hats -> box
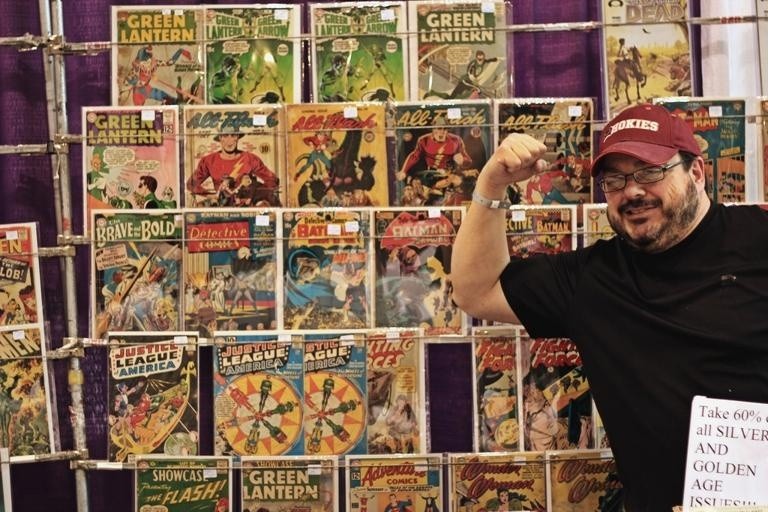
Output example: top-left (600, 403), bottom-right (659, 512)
top-left (590, 104), bottom-right (701, 177)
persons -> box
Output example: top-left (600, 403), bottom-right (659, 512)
top-left (449, 101), bottom-right (768, 512)
top-left (0, 285), bottom-right (39, 326)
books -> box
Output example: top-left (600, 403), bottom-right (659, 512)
top-left (81, 0), bottom-right (745, 512)
top-left (0, 321), bottom-right (63, 466)
top-left (0, 219), bottom-right (45, 327)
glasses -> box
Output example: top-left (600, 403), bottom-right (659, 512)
top-left (596, 159), bottom-right (684, 193)
